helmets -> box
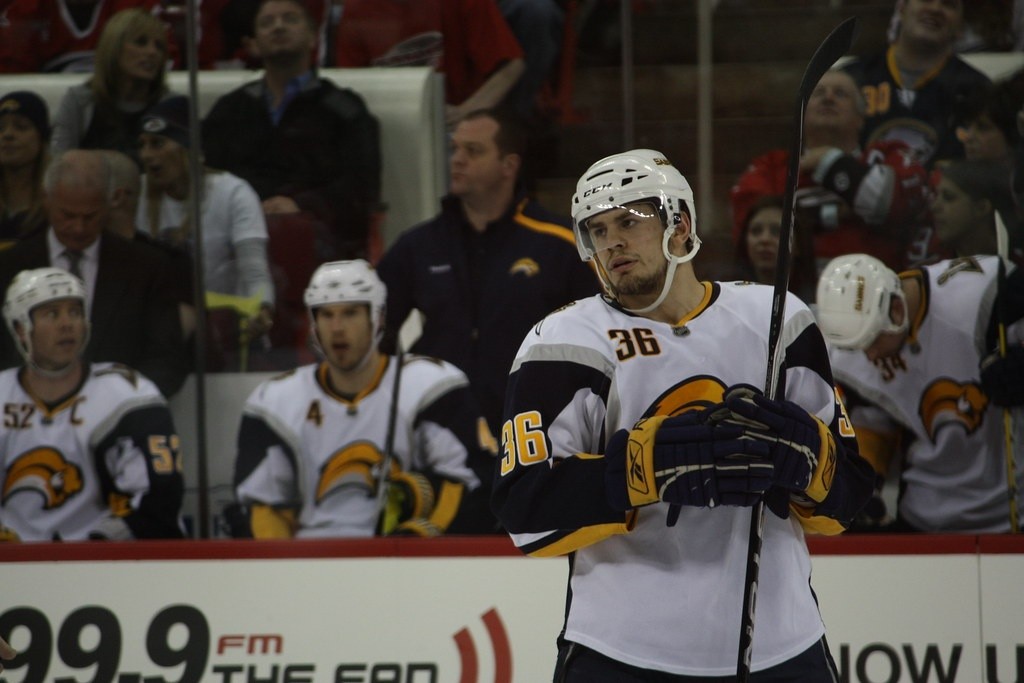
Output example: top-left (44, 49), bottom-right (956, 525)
top-left (816, 254), bottom-right (909, 349)
top-left (3, 266), bottom-right (90, 360)
top-left (571, 149), bottom-right (694, 261)
top-left (303, 259), bottom-right (386, 337)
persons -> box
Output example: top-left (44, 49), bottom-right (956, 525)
top-left (727, 0), bottom-right (1024, 537)
top-left (233, 259), bottom-right (497, 543)
top-left (0, 268), bottom-right (184, 546)
top-left (490, 149), bottom-right (874, 683)
top-left (0, 0), bottom-right (326, 393)
top-left (376, 109), bottom-right (601, 417)
top-left (201, 0), bottom-right (383, 370)
top-left (333, 0), bottom-right (526, 143)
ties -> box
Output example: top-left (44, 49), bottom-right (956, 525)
top-left (63, 249), bottom-right (81, 280)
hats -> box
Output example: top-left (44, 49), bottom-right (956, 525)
top-left (137, 95), bottom-right (190, 145)
top-left (0, 91), bottom-right (48, 137)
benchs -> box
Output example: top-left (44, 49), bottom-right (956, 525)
top-left (0, 48), bottom-right (1024, 540)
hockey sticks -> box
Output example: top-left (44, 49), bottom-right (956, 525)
top-left (992, 209), bottom-right (1019, 532)
top-left (736, 16), bottom-right (858, 682)
top-left (374, 307), bottom-right (424, 540)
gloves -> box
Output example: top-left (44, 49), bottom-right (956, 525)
top-left (817, 150), bottom-right (891, 218)
top-left (979, 322), bottom-right (1024, 406)
top-left (605, 401), bottom-right (774, 527)
top-left (716, 384), bottom-right (836, 506)
